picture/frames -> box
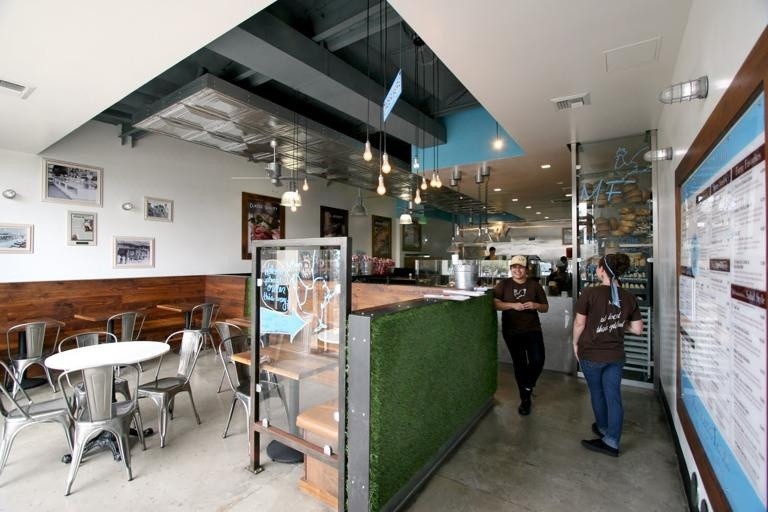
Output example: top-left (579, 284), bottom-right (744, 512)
top-left (66, 209), bottom-right (99, 247)
top-left (368, 214), bottom-right (392, 260)
top-left (0, 222), bottom-right (34, 255)
top-left (238, 190), bottom-right (288, 262)
top-left (401, 218), bottom-right (423, 253)
top-left (142, 195), bottom-right (174, 224)
top-left (318, 204), bottom-right (350, 250)
top-left (39, 156), bottom-right (104, 209)
top-left (109, 234), bottom-right (156, 269)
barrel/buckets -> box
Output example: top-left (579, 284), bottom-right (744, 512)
top-left (352, 261), bottom-right (361, 277)
top-left (453, 263), bottom-right (479, 291)
top-left (360, 260), bottom-right (376, 275)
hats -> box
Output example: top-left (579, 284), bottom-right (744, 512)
top-left (509, 255), bottom-right (527, 267)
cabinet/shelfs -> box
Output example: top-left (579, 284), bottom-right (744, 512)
top-left (578, 201), bottom-right (652, 307)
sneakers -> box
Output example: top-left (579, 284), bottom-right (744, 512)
top-left (592, 421), bottom-right (605, 437)
top-left (518, 386), bottom-right (533, 416)
top-left (581, 438), bottom-right (619, 458)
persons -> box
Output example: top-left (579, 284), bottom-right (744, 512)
top-left (493, 255), bottom-right (549, 416)
top-left (485, 247), bottom-right (497, 260)
top-left (546, 256), bottom-right (573, 296)
top-left (572, 254), bottom-right (643, 458)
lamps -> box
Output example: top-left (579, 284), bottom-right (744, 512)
top-left (656, 72), bottom-right (710, 106)
top-left (399, 201), bottom-right (428, 225)
top-left (494, 188), bottom-right (502, 192)
top-left (450, 166), bottom-right (462, 242)
top-left (279, 116), bottom-right (302, 213)
top-left (476, 162), bottom-right (490, 237)
top-left (469, 209), bottom-right (473, 223)
top-left (642, 146), bottom-right (673, 163)
top-left (350, 188), bottom-right (367, 217)
top-left (302, 122), bottom-right (309, 192)
top-left (493, 138), bottom-right (503, 149)
top-left (363, 2), bottom-right (373, 161)
top-left (381, 2), bottom-right (392, 174)
top-left (413, 34), bottom-right (443, 205)
top-left (377, 2), bottom-right (387, 197)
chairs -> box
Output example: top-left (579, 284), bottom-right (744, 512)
top-left (219, 334), bottom-right (291, 457)
top-left (1, 358), bottom-right (75, 468)
top-left (1, 318), bottom-right (66, 401)
top-left (57, 331), bottom-right (131, 418)
top-left (164, 301), bottom-right (222, 356)
top-left (57, 361), bottom-right (147, 497)
top-left (107, 309), bottom-right (147, 343)
top-left (214, 321), bottom-right (251, 394)
